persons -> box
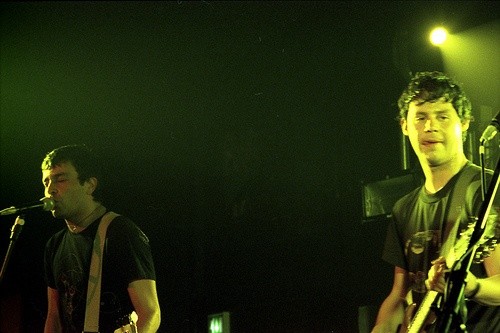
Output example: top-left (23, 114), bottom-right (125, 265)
top-left (36, 145), bottom-right (162, 333)
top-left (369, 69), bottom-right (500, 333)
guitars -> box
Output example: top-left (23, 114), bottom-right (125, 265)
top-left (397, 213), bottom-right (500, 333)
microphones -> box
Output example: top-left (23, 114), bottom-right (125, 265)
top-left (0, 197), bottom-right (56, 217)
top-left (478, 109), bottom-right (500, 142)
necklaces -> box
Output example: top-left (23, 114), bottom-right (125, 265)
top-left (64, 204), bottom-right (103, 231)
